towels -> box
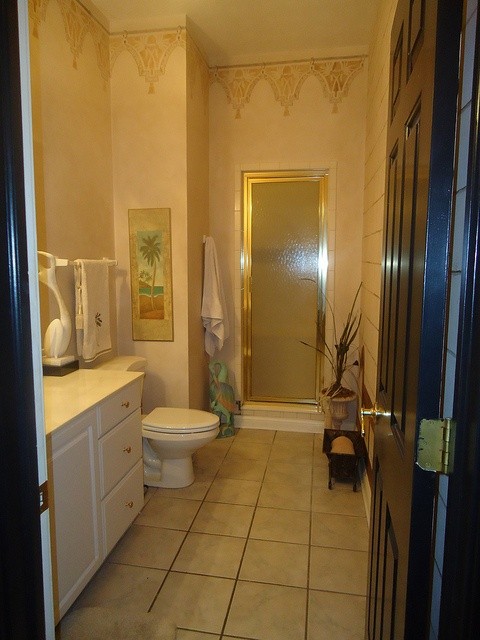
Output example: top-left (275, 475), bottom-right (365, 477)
top-left (74, 259), bottom-right (113, 363)
top-left (200, 235), bottom-right (230, 358)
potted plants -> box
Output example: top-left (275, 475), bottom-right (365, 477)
top-left (295, 277), bottom-right (363, 430)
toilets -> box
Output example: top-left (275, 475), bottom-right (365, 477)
top-left (94, 355), bottom-right (220, 489)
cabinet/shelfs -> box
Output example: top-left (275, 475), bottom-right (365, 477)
top-left (96, 381), bottom-right (144, 555)
top-left (46, 404), bottom-right (105, 629)
